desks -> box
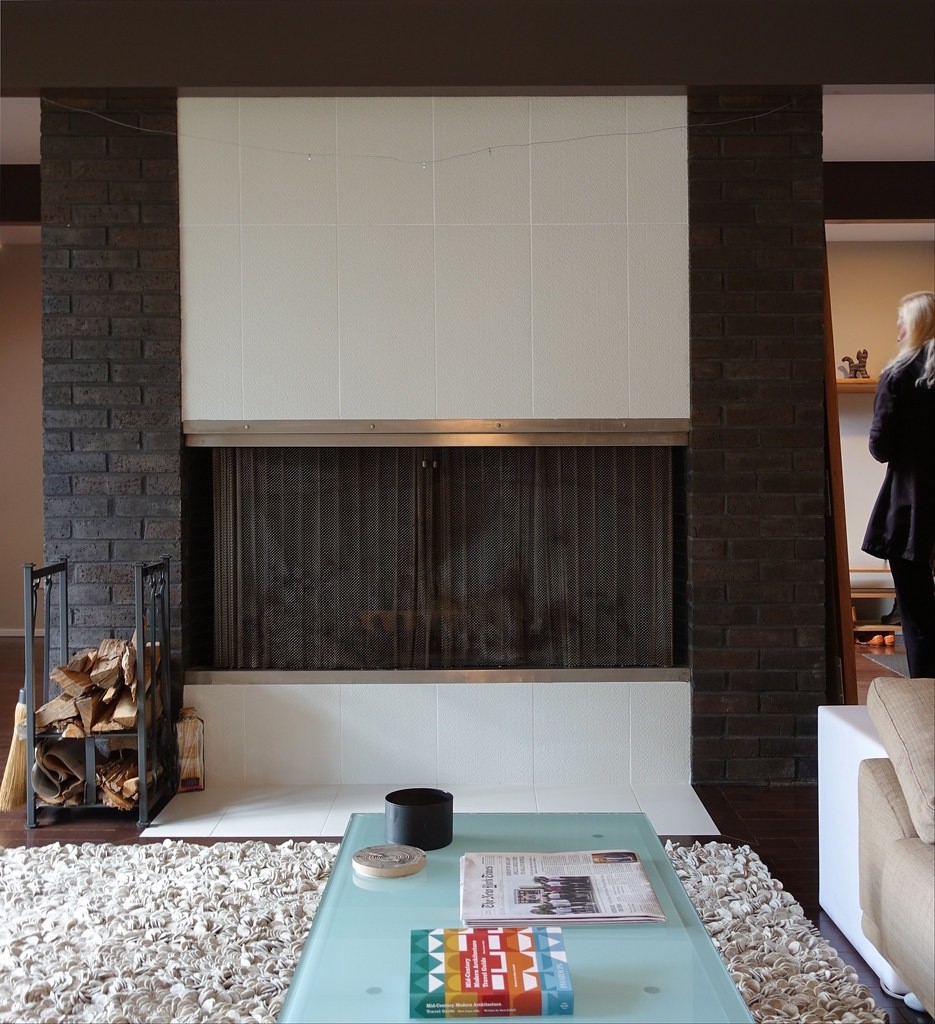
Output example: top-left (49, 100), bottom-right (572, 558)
top-left (279, 812), bottom-right (754, 1024)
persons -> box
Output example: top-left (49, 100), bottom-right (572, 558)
top-left (861, 292), bottom-right (935, 678)
top-left (593, 853), bottom-right (636, 863)
top-left (522, 876), bottom-right (595, 914)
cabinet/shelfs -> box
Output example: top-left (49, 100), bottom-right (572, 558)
top-left (21, 553), bottom-right (172, 828)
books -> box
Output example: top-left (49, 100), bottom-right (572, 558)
top-left (409, 926), bottom-right (574, 1019)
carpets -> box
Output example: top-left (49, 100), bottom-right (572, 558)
top-left (0, 839), bottom-right (890, 1024)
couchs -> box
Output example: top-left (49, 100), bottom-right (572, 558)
top-left (817, 678), bottom-right (935, 1020)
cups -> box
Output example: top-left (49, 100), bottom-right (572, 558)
top-left (383, 787), bottom-right (453, 851)
